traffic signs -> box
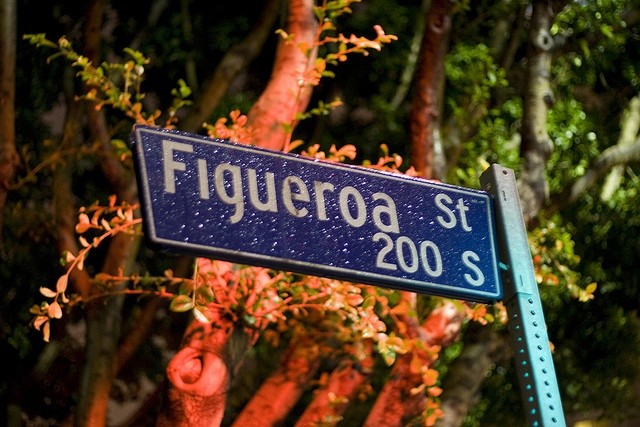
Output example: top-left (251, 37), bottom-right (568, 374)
top-left (131, 124), bottom-right (504, 303)
